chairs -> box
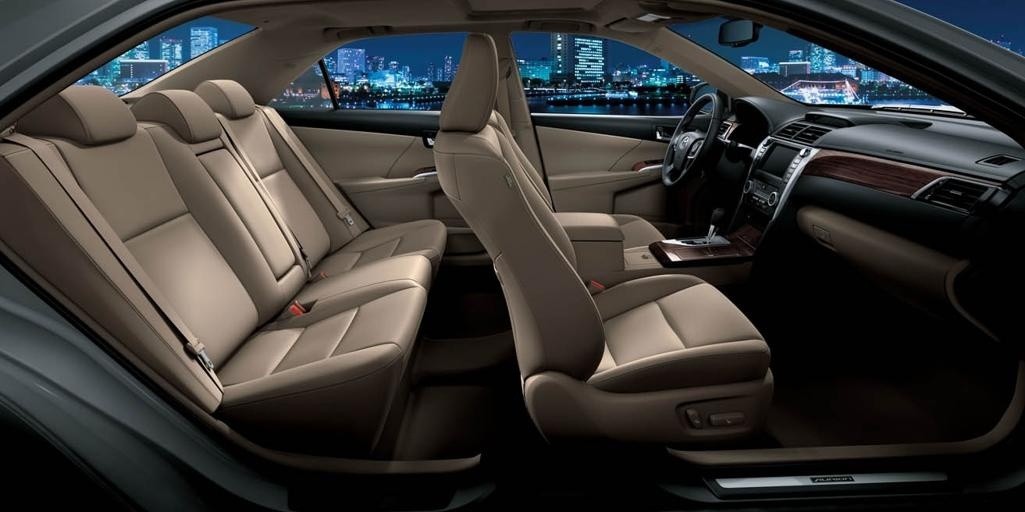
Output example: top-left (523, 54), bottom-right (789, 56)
top-left (194, 79), bottom-right (448, 274)
top-left (490, 32), bottom-right (696, 294)
top-left (430, 35), bottom-right (777, 448)
top-left (0, 82), bottom-right (428, 452)
top-left (130, 89), bottom-right (433, 332)
